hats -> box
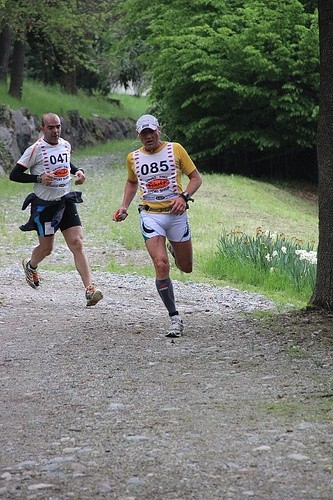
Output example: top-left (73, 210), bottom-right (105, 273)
top-left (135, 114), bottom-right (158, 134)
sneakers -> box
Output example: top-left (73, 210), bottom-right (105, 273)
top-left (22, 257), bottom-right (39, 289)
top-left (167, 242), bottom-right (173, 258)
top-left (85, 290), bottom-right (102, 306)
top-left (164, 316), bottom-right (183, 337)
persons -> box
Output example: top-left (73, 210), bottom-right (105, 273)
top-left (9, 113), bottom-right (103, 306)
top-left (114, 115), bottom-right (203, 336)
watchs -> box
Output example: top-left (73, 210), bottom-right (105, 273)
top-left (181, 192), bottom-right (190, 199)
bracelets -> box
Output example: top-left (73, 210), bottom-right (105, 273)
top-left (37, 176), bottom-right (41, 182)
